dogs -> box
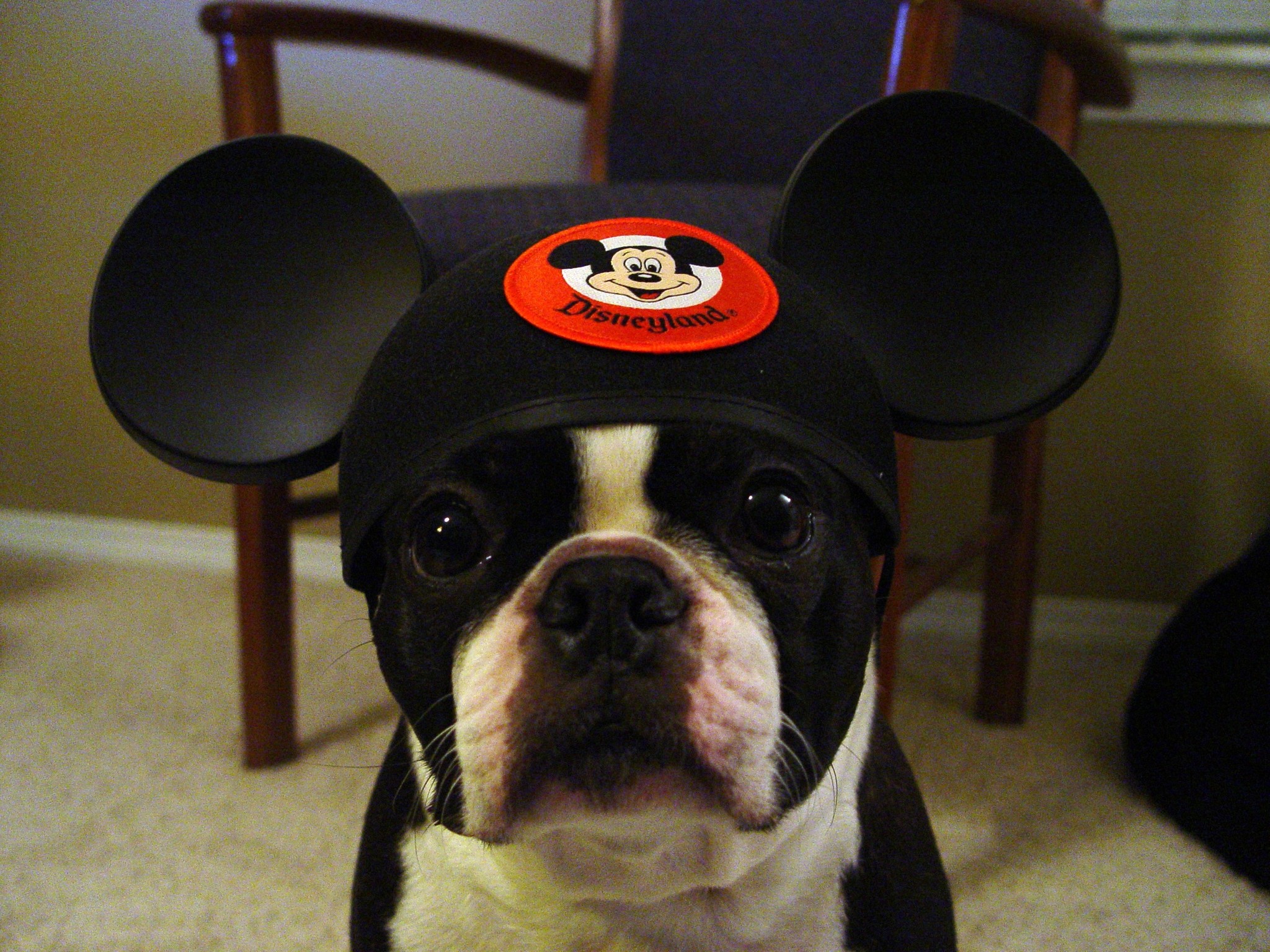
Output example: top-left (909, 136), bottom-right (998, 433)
top-left (348, 421), bottom-right (959, 952)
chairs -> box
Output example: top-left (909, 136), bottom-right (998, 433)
top-left (192, 2), bottom-right (1140, 782)
